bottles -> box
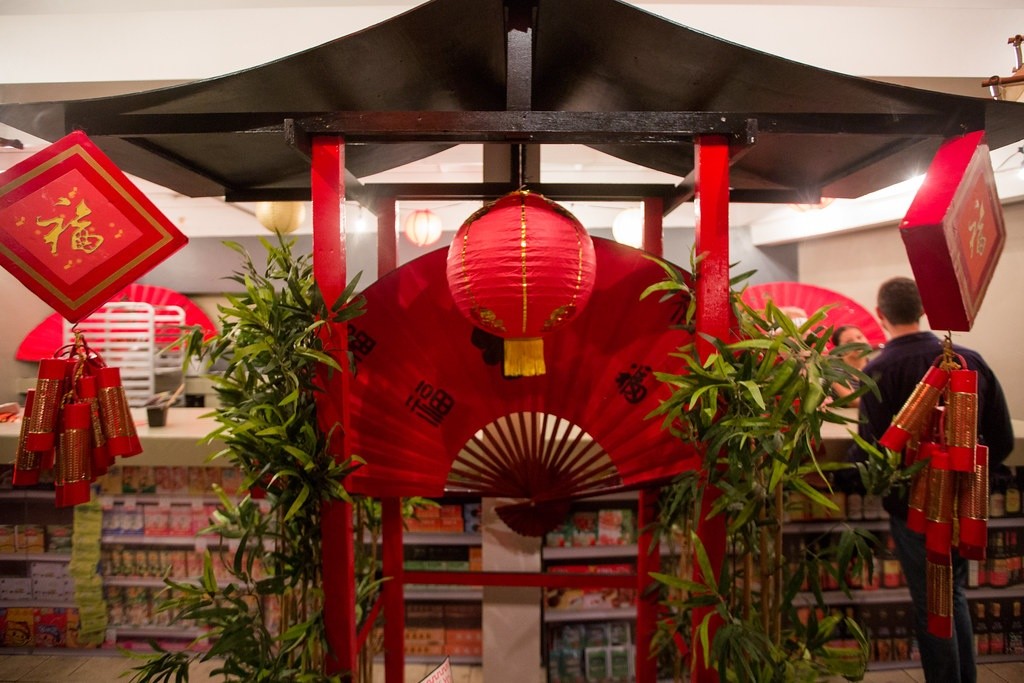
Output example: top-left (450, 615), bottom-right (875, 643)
top-left (769, 533), bottom-right (1024, 665)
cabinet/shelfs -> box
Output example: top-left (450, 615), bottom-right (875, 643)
top-left (0, 489), bottom-right (1024, 665)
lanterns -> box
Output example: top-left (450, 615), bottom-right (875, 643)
top-left (447, 191), bottom-right (596, 376)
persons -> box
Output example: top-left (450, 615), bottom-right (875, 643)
top-left (858, 277), bottom-right (1014, 683)
top-left (829, 326), bottom-right (871, 408)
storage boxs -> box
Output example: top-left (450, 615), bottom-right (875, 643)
top-left (0, 467), bottom-right (635, 682)
